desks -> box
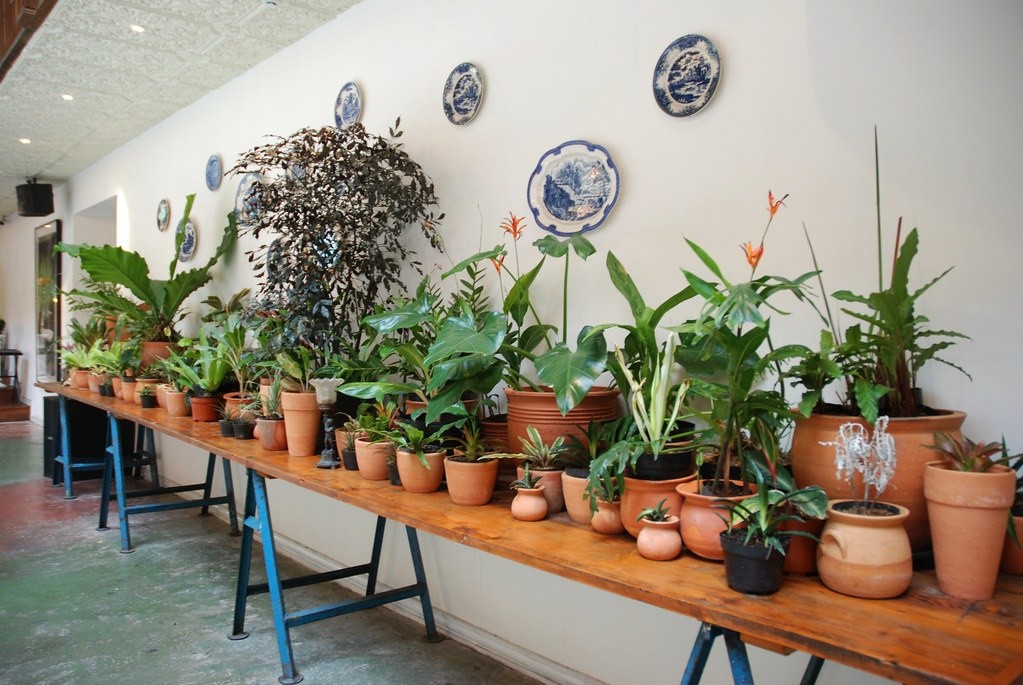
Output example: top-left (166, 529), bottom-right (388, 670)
top-left (0, 349), bottom-right (23, 406)
top-left (35, 382), bottom-right (1023, 685)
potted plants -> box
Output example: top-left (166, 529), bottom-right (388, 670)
top-left (41, 118), bottom-right (1023, 598)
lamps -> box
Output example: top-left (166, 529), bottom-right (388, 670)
top-left (15, 177), bottom-right (53, 218)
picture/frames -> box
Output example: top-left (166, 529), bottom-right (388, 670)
top-left (33, 220), bottom-right (62, 384)
